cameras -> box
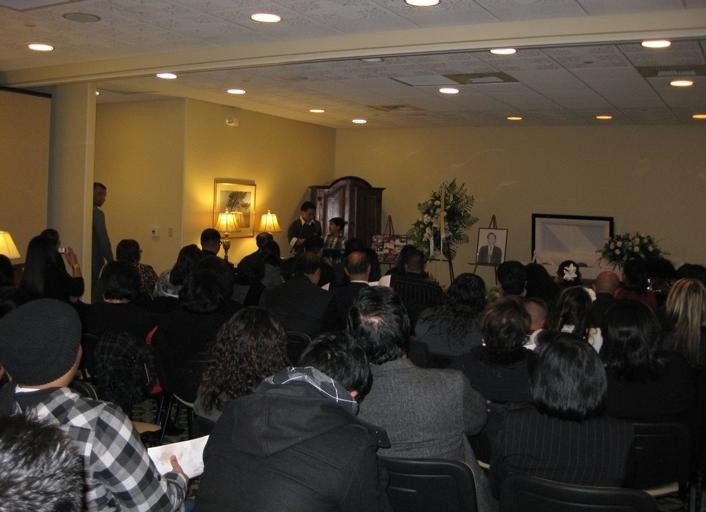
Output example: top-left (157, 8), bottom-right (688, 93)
top-left (57, 247), bottom-right (68, 254)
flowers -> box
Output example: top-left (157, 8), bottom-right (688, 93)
top-left (595, 232), bottom-right (671, 272)
top-left (407, 178), bottom-right (479, 263)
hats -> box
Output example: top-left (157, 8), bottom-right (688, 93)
top-left (1, 299), bottom-right (83, 386)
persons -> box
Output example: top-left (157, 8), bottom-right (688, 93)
top-left (324, 217), bottom-right (346, 276)
top-left (289, 201), bottom-right (323, 256)
top-left (1, 228), bottom-right (706, 511)
top-left (92, 183), bottom-right (115, 295)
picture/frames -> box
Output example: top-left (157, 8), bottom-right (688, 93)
top-left (477, 227), bottom-right (508, 264)
top-left (531, 213), bottom-right (614, 266)
top-left (213, 178), bottom-right (256, 240)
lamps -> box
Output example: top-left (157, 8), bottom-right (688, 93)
top-left (258, 210), bottom-right (282, 234)
top-left (0, 230), bottom-right (21, 259)
top-left (215, 210), bottom-right (241, 261)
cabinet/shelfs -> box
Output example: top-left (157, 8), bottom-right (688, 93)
top-left (309, 176), bottom-right (386, 244)
